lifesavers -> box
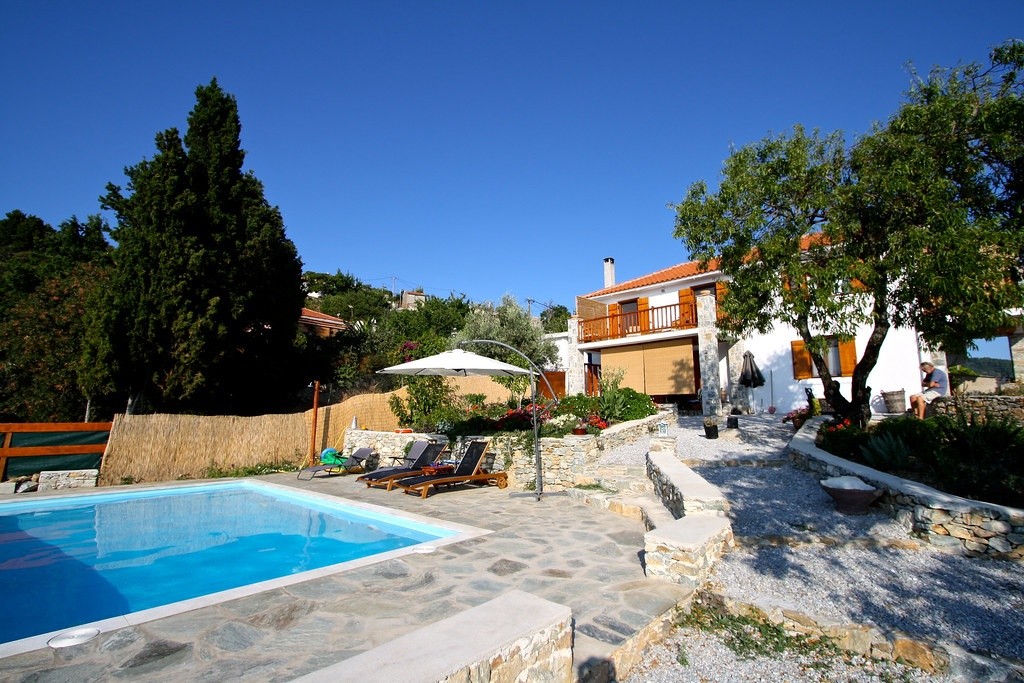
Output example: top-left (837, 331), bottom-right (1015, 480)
top-left (321, 448), bottom-right (336, 460)
top-left (396, 429), bottom-right (412, 433)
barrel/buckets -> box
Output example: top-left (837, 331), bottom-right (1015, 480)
top-left (880, 387), bottom-right (906, 413)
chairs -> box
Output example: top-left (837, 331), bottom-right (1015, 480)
top-left (368, 441), bottom-right (428, 474)
top-left (297, 448), bottom-right (375, 481)
top-left (355, 443), bottom-right (454, 490)
top-left (393, 441), bottom-right (508, 499)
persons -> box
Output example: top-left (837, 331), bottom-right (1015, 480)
top-left (910, 362), bottom-right (948, 418)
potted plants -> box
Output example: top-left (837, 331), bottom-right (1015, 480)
top-left (702, 416), bottom-right (719, 438)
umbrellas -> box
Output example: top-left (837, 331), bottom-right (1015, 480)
top-left (739, 350), bottom-right (766, 413)
top-left (376, 348), bottom-right (540, 378)
